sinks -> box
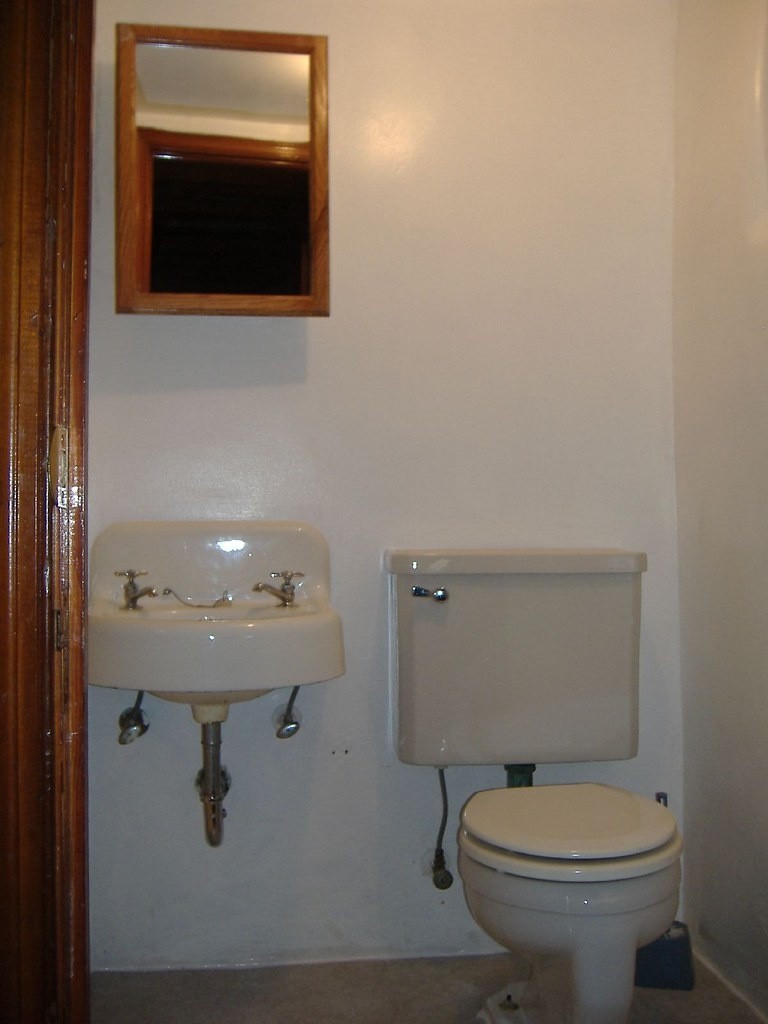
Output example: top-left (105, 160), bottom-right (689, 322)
top-left (88, 607), bottom-right (347, 692)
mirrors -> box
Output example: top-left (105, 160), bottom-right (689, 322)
top-left (114, 22), bottom-right (330, 318)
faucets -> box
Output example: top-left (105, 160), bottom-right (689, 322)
top-left (252, 569), bottom-right (305, 609)
top-left (114, 568), bottom-right (159, 612)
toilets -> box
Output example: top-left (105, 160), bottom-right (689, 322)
top-left (383, 547), bottom-right (685, 1023)
